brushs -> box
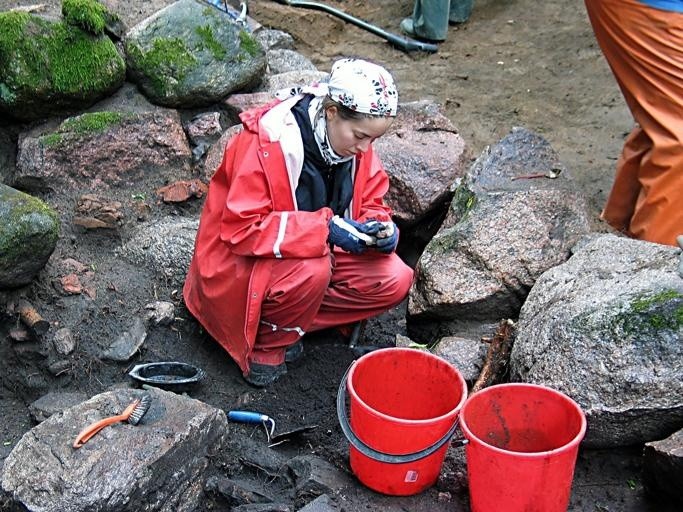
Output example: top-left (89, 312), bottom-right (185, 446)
top-left (73, 392), bottom-right (152, 448)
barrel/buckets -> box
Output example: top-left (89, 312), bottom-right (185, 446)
top-left (334, 347), bottom-right (464, 496)
top-left (459, 383), bottom-right (589, 512)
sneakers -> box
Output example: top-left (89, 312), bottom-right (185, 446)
top-left (285, 338), bottom-right (303, 370)
top-left (244, 362), bottom-right (289, 387)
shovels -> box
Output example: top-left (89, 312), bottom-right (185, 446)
top-left (227, 411), bottom-right (320, 441)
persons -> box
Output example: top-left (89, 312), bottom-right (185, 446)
top-left (180, 58), bottom-right (415, 387)
top-left (402, 1), bottom-right (472, 44)
top-left (583, 1), bottom-right (683, 248)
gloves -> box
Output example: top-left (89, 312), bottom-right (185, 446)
top-left (327, 213), bottom-right (375, 254)
top-left (365, 220), bottom-right (399, 256)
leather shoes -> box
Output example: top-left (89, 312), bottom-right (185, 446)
top-left (398, 15), bottom-right (445, 43)
top-left (447, 15), bottom-right (465, 26)
